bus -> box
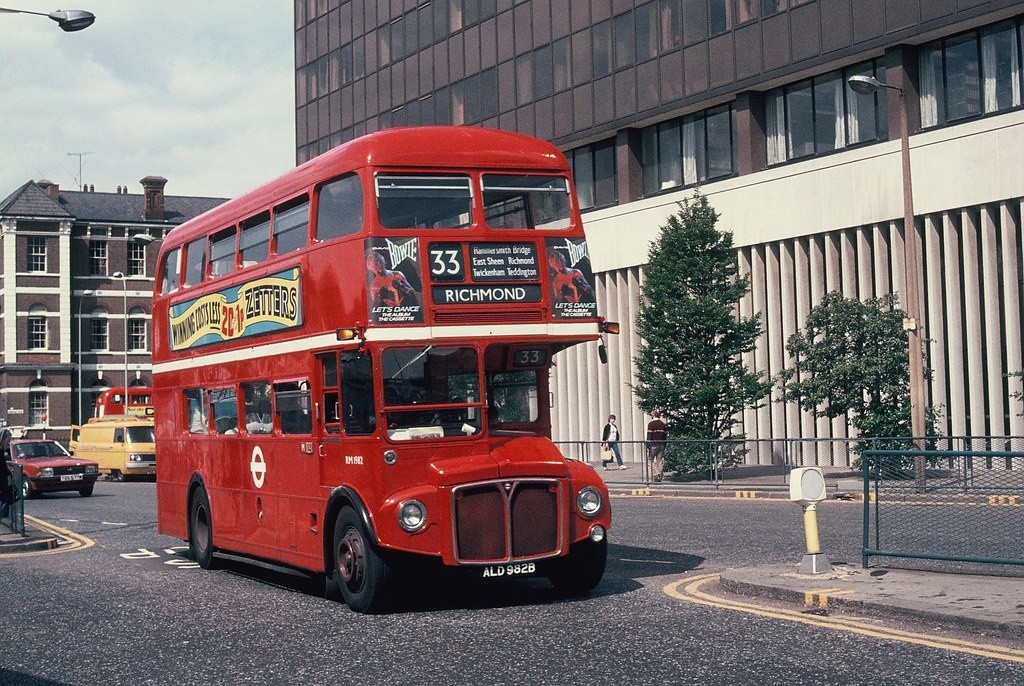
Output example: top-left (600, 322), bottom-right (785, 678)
top-left (21, 422), bottom-right (74, 455)
top-left (154, 120), bottom-right (619, 618)
top-left (95, 386), bottom-right (153, 419)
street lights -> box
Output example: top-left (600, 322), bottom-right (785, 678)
top-left (78, 289), bottom-right (93, 427)
top-left (848, 76), bottom-right (929, 492)
top-left (111, 270), bottom-right (129, 416)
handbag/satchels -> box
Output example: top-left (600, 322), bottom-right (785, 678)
top-left (601, 446), bottom-right (612, 461)
top-left (6, 471), bottom-right (21, 505)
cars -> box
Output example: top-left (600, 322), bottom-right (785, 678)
top-left (1, 423), bottom-right (28, 450)
top-left (10, 438), bottom-right (102, 499)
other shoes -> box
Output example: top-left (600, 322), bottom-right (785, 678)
top-left (603, 467), bottom-right (608, 471)
top-left (619, 465), bottom-right (627, 470)
top-left (652, 474), bottom-right (661, 482)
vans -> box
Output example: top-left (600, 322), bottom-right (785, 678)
top-left (67, 414), bottom-right (159, 483)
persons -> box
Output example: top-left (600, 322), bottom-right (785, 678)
top-left (646, 409), bottom-right (668, 481)
top-left (19, 428), bottom-right (29, 439)
top-left (603, 415), bottom-right (629, 471)
top-left (0, 429), bottom-right (12, 520)
